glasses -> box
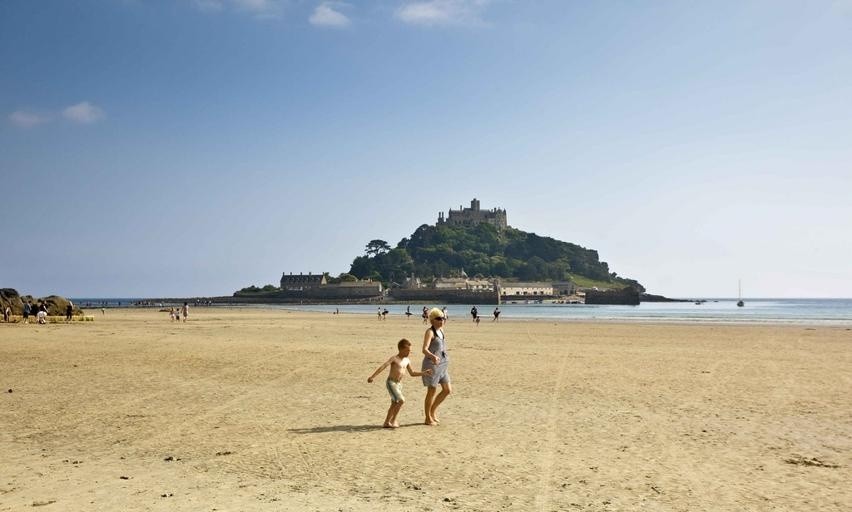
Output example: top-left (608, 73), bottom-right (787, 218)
top-left (435, 317), bottom-right (446, 321)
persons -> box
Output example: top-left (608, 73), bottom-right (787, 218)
top-left (175, 308), bottom-right (182, 321)
top-left (23, 299), bottom-right (50, 324)
top-left (5, 305), bottom-right (14, 323)
top-left (375, 302), bottom-right (503, 327)
top-left (169, 308), bottom-right (176, 320)
top-left (65, 300), bottom-right (74, 321)
top-left (180, 302), bottom-right (190, 323)
top-left (367, 339), bottom-right (434, 428)
top-left (420, 308), bottom-right (452, 426)
